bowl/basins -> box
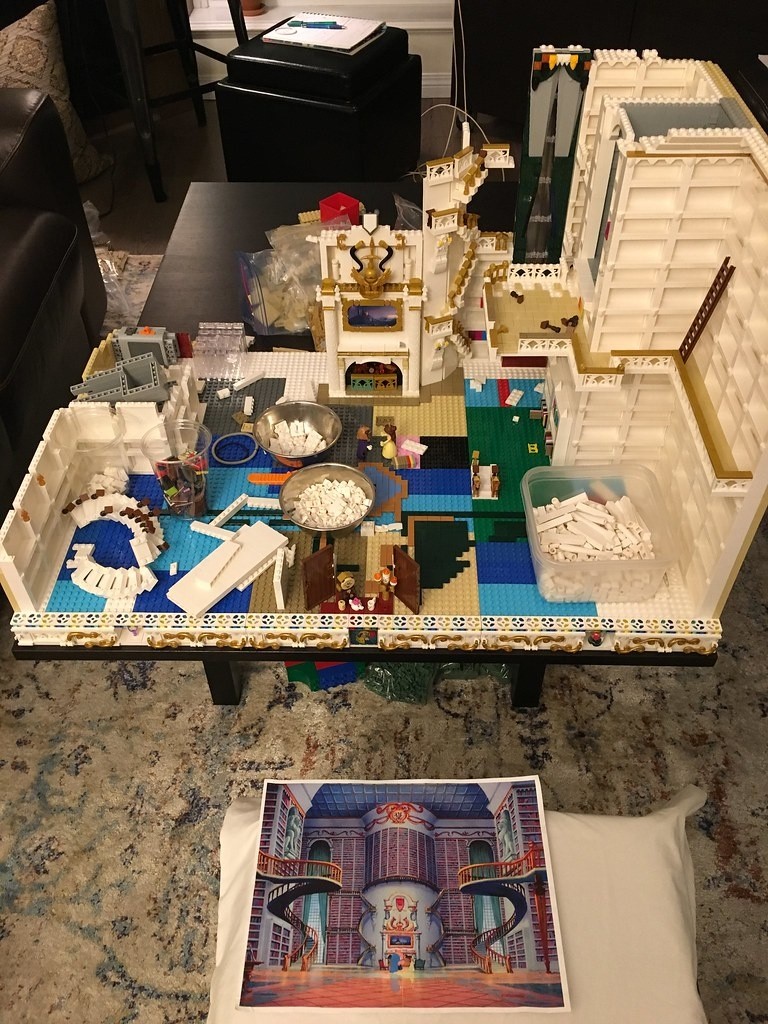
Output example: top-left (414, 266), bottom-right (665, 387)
top-left (253, 400), bottom-right (342, 467)
top-left (279, 462), bottom-right (376, 538)
top-left (519, 464), bottom-right (676, 602)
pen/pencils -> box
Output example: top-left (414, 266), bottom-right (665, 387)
top-left (301, 21), bottom-right (337, 25)
top-left (301, 24), bottom-right (347, 29)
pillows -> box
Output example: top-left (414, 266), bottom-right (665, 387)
top-left (0, 0), bottom-right (106, 184)
top-left (205, 784), bottom-right (709, 1024)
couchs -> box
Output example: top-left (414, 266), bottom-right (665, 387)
top-left (0, 87), bottom-right (108, 608)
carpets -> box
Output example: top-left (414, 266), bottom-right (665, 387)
top-left (321, 591), bottom-right (394, 614)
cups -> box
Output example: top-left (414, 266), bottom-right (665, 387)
top-left (139, 419), bottom-right (212, 521)
top-left (54, 406), bottom-right (133, 500)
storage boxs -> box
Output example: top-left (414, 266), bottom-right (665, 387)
top-left (519, 465), bottom-right (679, 604)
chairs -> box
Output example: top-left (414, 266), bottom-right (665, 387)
top-left (379, 959), bottom-right (389, 970)
top-left (414, 959), bottom-right (426, 970)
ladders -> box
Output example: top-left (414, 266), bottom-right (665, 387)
top-left (679, 256), bottom-right (736, 364)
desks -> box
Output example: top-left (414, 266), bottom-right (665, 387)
top-left (541, 320), bottom-right (561, 332)
top-left (510, 291), bottom-right (524, 305)
top-left (561, 315), bottom-right (580, 326)
top-left (121, 181), bottom-right (717, 709)
